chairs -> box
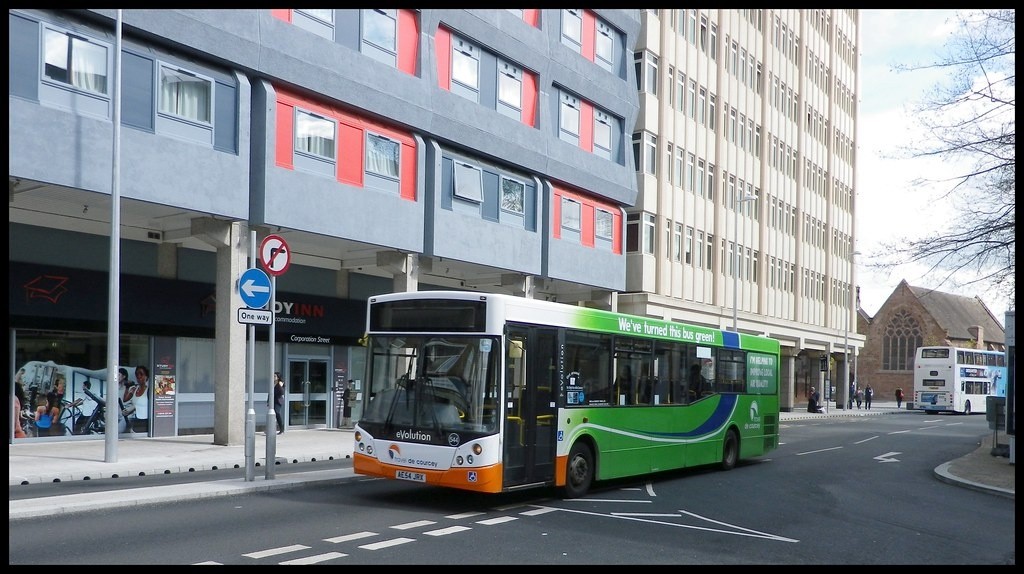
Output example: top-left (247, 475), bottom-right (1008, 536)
top-left (633, 376), bottom-right (653, 405)
top-left (688, 390), bottom-right (697, 403)
top-left (654, 377), bottom-right (676, 405)
top-left (734, 379), bottom-right (744, 394)
top-left (718, 380), bottom-right (734, 393)
top-left (700, 391), bottom-right (715, 399)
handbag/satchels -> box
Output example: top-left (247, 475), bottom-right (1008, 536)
top-left (900, 389), bottom-right (904, 399)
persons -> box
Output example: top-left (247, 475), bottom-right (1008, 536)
top-left (14, 366), bottom-right (149, 438)
top-left (267, 372), bottom-right (285, 435)
top-left (613, 363), bottom-right (706, 404)
top-left (809, 384), bottom-right (904, 413)
top-left (341, 380), bottom-right (354, 427)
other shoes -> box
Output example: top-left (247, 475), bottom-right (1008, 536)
top-left (277, 431), bottom-right (284, 435)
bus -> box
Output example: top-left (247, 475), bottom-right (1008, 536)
top-left (913, 346), bottom-right (1007, 415)
top-left (352, 290), bottom-right (781, 500)
